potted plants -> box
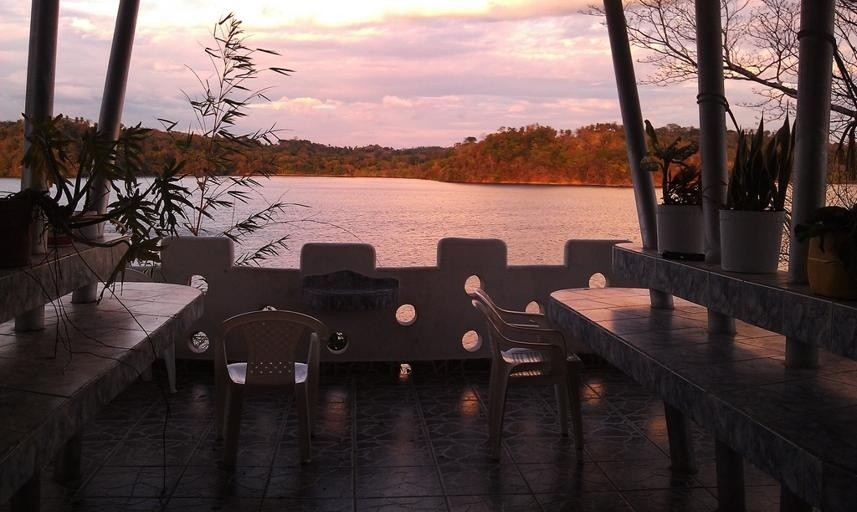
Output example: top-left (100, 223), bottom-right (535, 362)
top-left (639, 116), bottom-right (856, 299)
top-left (0, 114), bottom-right (188, 305)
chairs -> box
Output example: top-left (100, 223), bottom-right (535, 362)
top-left (208, 308), bottom-right (327, 471)
top-left (470, 285), bottom-right (588, 467)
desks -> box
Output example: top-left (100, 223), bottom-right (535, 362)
top-left (0, 234), bottom-right (208, 511)
top-left (547, 241), bottom-right (856, 511)
